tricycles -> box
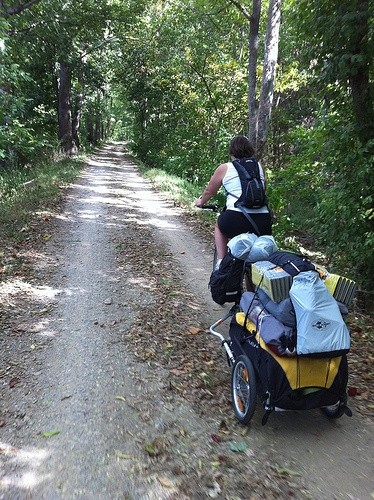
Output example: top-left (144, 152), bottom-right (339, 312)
top-left (197, 203), bottom-right (347, 424)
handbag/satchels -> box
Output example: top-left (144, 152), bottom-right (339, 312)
top-left (227, 232), bottom-right (352, 356)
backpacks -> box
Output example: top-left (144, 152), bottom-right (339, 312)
top-left (231, 157), bottom-right (267, 209)
top-left (209, 253), bottom-right (246, 306)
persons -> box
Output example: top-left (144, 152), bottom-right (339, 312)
top-left (194, 136), bottom-right (272, 272)
